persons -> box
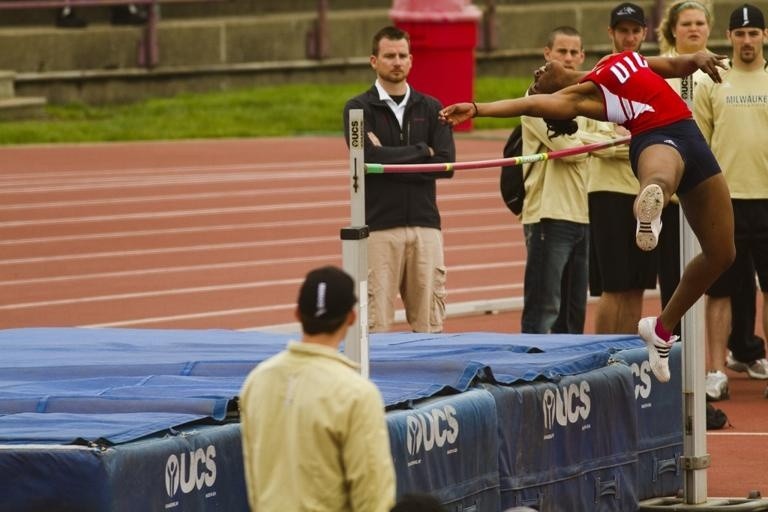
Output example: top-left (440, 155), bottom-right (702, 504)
top-left (237, 264), bottom-right (398, 511)
top-left (343, 26), bottom-right (455, 335)
top-left (438, 0), bottom-right (766, 433)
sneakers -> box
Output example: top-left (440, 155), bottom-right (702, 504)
top-left (634, 180), bottom-right (664, 249)
top-left (684, 346), bottom-right (767, 431)
top-left (636, 314), bottom-right (681, 385)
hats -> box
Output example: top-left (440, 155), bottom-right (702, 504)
top-left (609, 3), bottom-right (647, 29)
top-left (729, 3), bottom-right (764, 30)
top-left (297, 267), bottom-right (359, 325)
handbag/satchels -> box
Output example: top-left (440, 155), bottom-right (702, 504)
top-left (499, 122), bottom-right (525, 217)
